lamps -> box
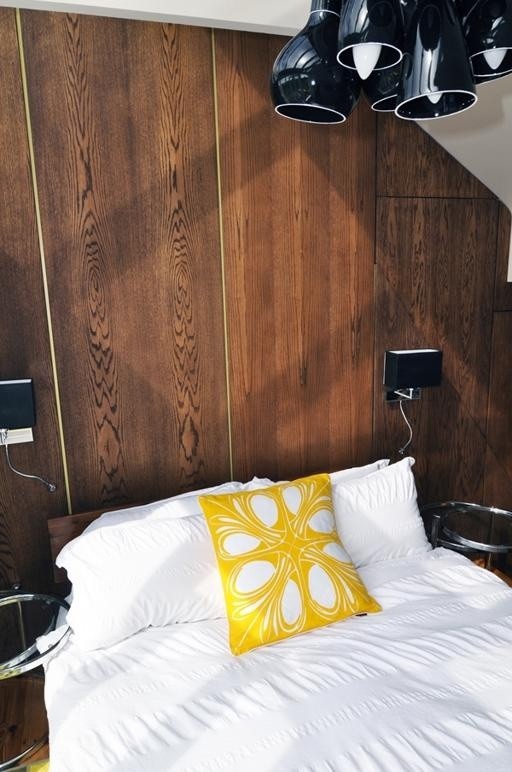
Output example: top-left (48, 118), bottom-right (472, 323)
top-left (267, 1), bottom-right (512, 125)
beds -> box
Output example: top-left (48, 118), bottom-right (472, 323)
top-left (36, 456), bottom-right (512, 772)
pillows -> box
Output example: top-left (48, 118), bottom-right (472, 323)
top-left (199, 473), bottom-right (383, 655)
top-left (56, 512), bottom-right (227, 652)
top-left (332, 456), bottom-right (432, 591)
